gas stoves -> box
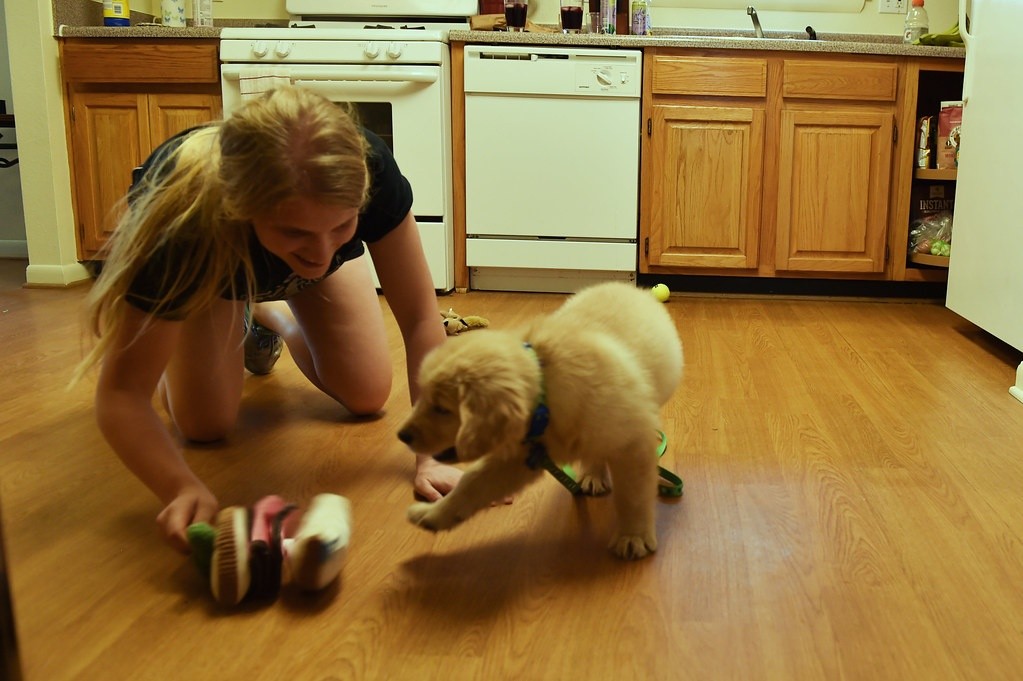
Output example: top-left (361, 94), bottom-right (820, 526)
top-left (218, 0), bottom-right (478, 63)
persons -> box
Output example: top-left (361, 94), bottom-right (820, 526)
top-left (69, 84), bottom-right (514, 553)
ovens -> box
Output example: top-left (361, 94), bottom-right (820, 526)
top-left (219, 64), bottom-right (452, 293)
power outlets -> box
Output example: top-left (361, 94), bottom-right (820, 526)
top-left (878, 0), bottom-right (907, 15)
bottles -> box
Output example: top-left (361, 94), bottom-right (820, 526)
top-left (192, 0), bottom-right (214, 26)
top-left (161, 0), bottom-right (186, 27)
top-left (903, 0), bottom-right (929, 45)
top-left (102, 0), bottom-right (131, 27)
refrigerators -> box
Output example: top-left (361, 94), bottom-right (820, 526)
top-left (946, 1), bottom-right (1023, 353)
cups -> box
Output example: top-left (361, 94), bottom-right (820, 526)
top-left (601, 0), bottom-right (617, 36)
top-left (503, 0), bottom-right (529, 32)
top-left (559, 0), bottom-right (584, 35)
top-left (587, 13), bottom-right (603, 34)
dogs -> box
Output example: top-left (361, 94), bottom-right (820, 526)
top-left (396, 280), bottom-right (684, 562)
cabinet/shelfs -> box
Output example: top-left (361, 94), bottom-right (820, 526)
top-left (57, 37), bottom-right (223, 264)
top-left (892, 57), bottom-right (965, 283)
top-left (639, 46), bottom-right (904, 283)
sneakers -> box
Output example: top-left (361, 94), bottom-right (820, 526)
top-left (244, 301), bottom-right (282, 374)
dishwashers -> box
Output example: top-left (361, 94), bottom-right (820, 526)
top-left (463, 46), bottom-right (643, 293)
